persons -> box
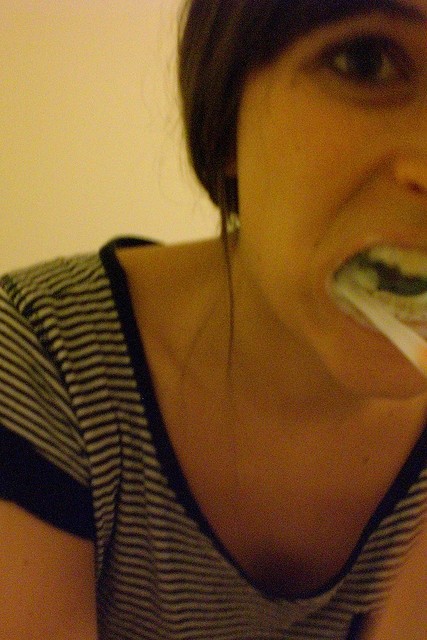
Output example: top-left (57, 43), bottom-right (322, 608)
top-left (0, 0), bottom-right (426, 633)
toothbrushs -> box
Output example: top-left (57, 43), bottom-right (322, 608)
top-left (337, 274), bottom-right (427, 379)
